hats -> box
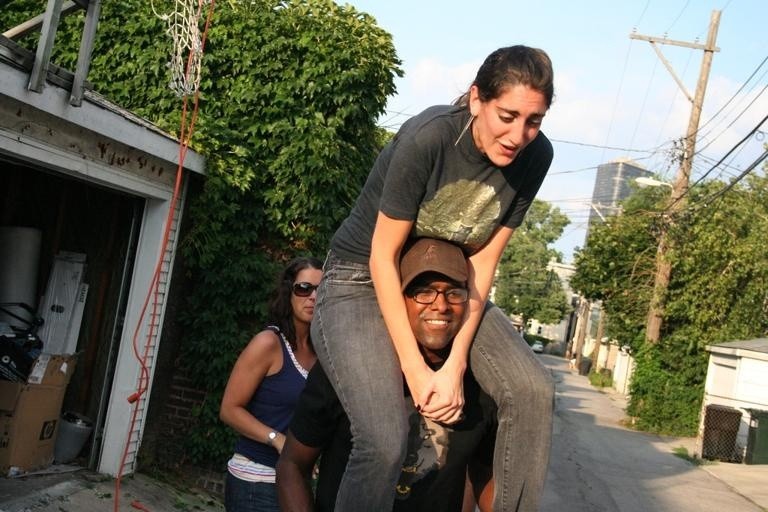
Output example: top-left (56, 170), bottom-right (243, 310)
top-left (400, 239), bottom-right (469, 295)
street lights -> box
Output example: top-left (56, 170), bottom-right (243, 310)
top-left (634, 176), bottom-right (687, 418)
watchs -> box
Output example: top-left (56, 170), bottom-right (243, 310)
top-left (268, 429), bottom-right (278, 447)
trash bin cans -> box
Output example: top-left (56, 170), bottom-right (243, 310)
top-left (702, 403), bottom-right (768, 465)
top-left (579, 358), bottom-right (592, 376)
top-left (54, 409), bottom-right (95, 464)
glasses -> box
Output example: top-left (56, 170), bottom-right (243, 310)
top-left (411, 288), bottom-right (470, 304)
top-left (289, 282), bottom-right (321, 297)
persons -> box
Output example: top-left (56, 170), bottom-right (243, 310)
top-left (308, 47), bottom-right (555, 512)
top-left (276, 239), bottom-right (497, 512)
top-left (219, 251), bottom-right (323, 512)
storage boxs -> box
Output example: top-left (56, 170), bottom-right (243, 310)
top-left (0, 354), bottom-right (80, 475)
